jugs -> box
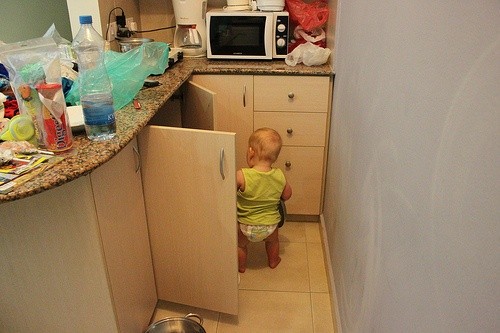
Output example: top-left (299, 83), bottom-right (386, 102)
top-left (171, 0), bottom-right (207, 57)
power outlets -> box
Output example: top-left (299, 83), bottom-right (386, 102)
top-left (107, 17), bottom-right (133, 41)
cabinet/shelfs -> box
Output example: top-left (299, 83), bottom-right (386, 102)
top-left (0, 67), bottom-right (336, 333)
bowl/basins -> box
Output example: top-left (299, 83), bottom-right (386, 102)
top-left (119, 38), bottom-right (155, 54)
top-left (144, 313), bottom-right (207, 333)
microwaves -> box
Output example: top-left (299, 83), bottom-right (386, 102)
top-left (205, 10), bottom-right (290, 60)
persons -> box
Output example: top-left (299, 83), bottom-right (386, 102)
top-left (236, 127), bottom-right (292, 273)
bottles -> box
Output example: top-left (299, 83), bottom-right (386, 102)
top-left (70, 15), bottom-right (117, 142)
